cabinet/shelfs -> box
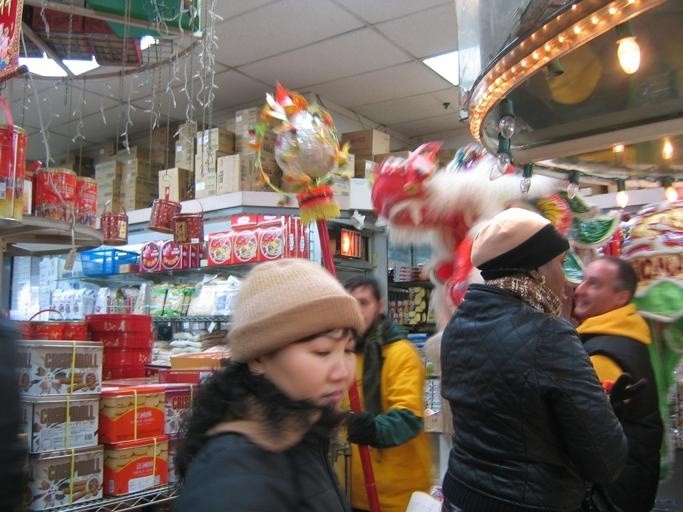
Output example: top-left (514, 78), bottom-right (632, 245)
top-left (0, 218), bottom-right (304, 511)
top-left (386, 272), bottom-right (442, 435)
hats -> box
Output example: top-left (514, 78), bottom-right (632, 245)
top-left (471, 207), bottom-right (570, 281)
top-left (226, 257), bottom-right (365, 367)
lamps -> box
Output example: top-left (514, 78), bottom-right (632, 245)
top-left (495, 22), bottom-right (678, 208)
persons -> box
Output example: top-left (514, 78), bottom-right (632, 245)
top-left (174, 258), bottom-right (362, 512)
top-left (573, 256), bottom-right (663, 512)
top-left (441, 207), bottom-right (630, 512)
top-left (324, 275), bottom-right (435, 512)
